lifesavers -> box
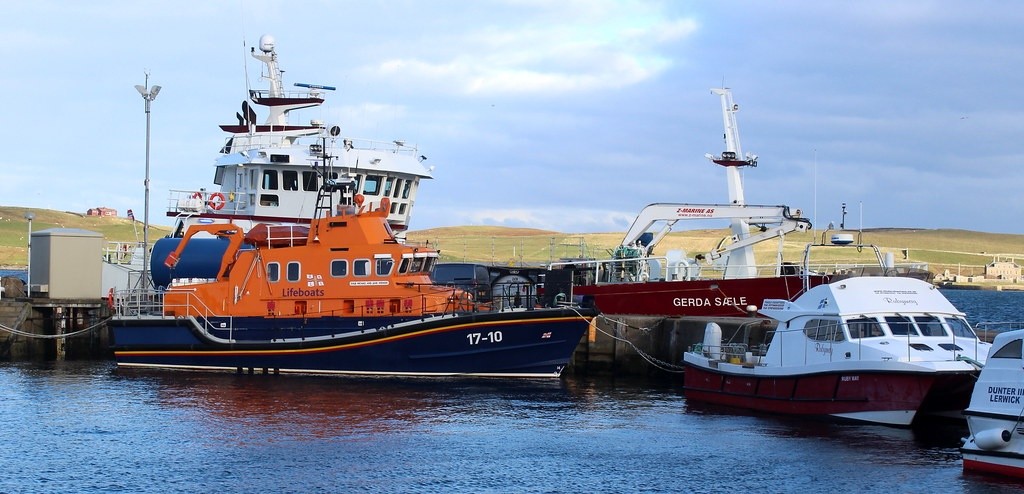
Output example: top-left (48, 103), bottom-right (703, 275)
top-left (108, 288), bottom-right (116, 308)
top-left (193, 192), bottom-right (202, 201)
top-left (209, 192), bottom-right (226, 210)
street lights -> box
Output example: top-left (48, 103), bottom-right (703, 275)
top-left (134, 85), bottom-right (162, 299)
top-left (24, 212), bottom-right (35, 297)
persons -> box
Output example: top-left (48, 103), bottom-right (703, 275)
top-left (630, 240), bottom-right (647, 259)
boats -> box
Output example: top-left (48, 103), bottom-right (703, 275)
top-left (112, 35), bottom-right (600, 378)
top-left (533, 76), bottom-right (934, 370)
top-left (957, 329), bottom-right (1024, 478)
top-left (684, 201), bottom-right (1000, 424)
